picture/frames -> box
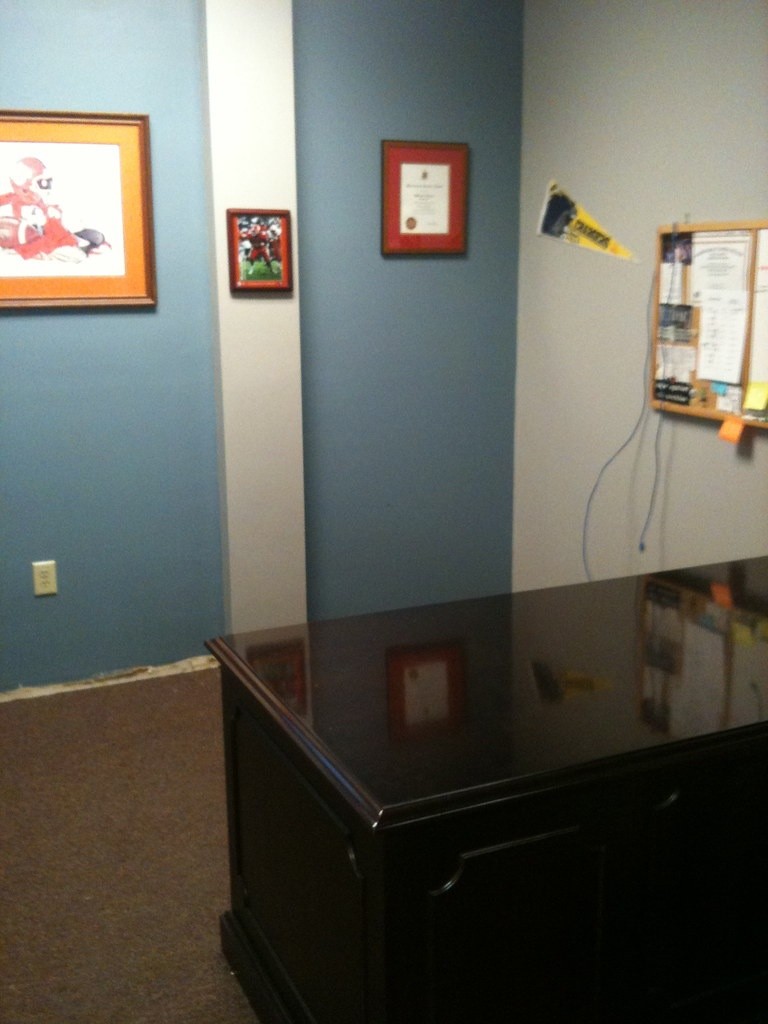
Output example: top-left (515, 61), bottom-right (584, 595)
top-left (227, 208), bottom-right (293, 292)
top-left (0, 109), bottom-right (159, 309)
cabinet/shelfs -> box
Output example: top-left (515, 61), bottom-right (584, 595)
top-left (205, 557), bottom-right (768, 1024)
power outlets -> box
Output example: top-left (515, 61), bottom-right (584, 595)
top-left (32, 560), bottom-right (57, 596)
